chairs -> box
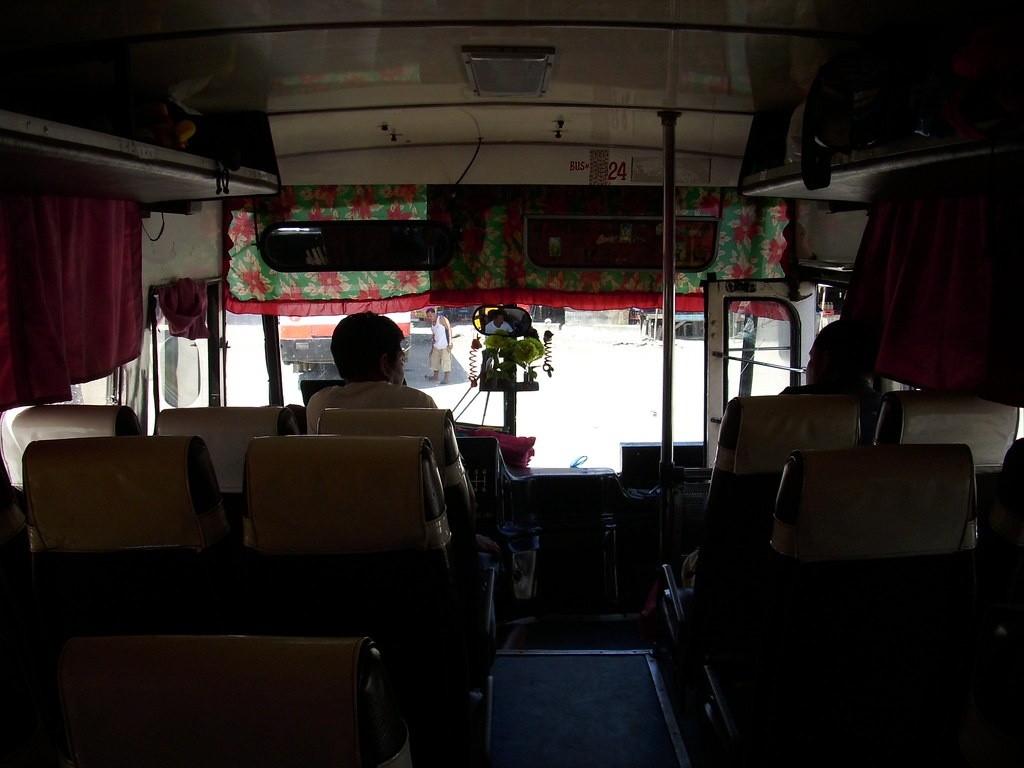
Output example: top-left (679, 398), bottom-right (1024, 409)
top-left (157, 406), bottom-right (300, 504)
top-left (58, 634), bottom-right (413, 768)
top-left (871, 389), bottom-right (1019, 472)
top-left (0, 454), bottom-right (26, 545)
top-left (22, 434), bottom-right (230, 591)
top-left (0, 404), bottom-right (144, 517)
top-left (698, 444), bottom-right (976, 749)
top-left (657, 394), bottom-right (861, 654)
top-left (316, 407), bottom-right (478, 546)
top-left (233, 434), bottom-right (453, 559)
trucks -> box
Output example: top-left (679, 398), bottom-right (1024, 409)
top-left (414, 305), bottom-right (569, 327)
top-left (278, 312), bottom-right (412, 390)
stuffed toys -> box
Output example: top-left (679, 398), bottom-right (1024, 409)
top-left (139, 70), bottom-right (197, 151)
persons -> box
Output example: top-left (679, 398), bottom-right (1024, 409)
top-left (780, 319), bottom-right (883, 447)
top-left (424, 308), bottom-right (453, 384)
top-left (484, 310), bottom-right (514, 334)
top-left (303, 311), bottom-right (502, 554)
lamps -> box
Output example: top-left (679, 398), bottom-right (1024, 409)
top-left (461, 45), bottom-right (557, 99)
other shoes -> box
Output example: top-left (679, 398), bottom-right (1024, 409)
top-left (440, 379), bottom-right (449, 384)
top-left (425, 375), bottom-right (438, 380)
